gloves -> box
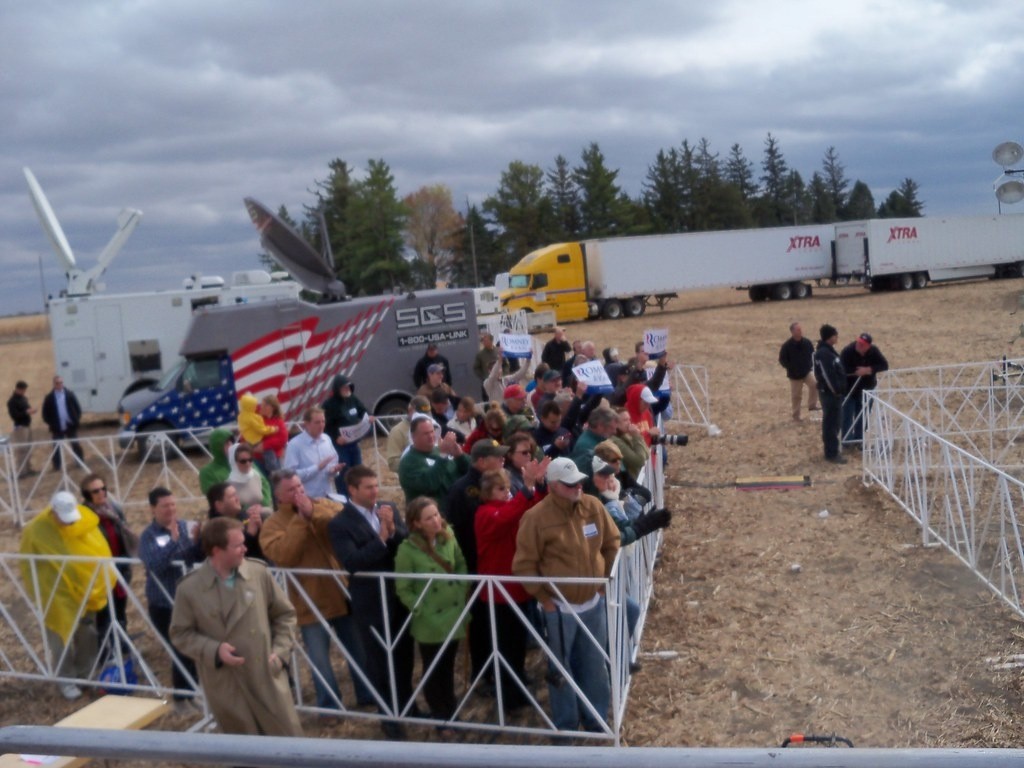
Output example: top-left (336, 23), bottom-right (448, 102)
top-left (631, 504), bottom-right (671, 540)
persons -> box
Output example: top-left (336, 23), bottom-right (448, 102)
top-left (138, 329), bottom-right (675, 743)
top-left (42, 376), bottom-right (84, 471)
top-left (20, 473), bottom-right (140, 698)
top-left (778, 322), bottom-right (821, 423)
top-left (7, 381), bottom-right (36, 478)
top-left (169, 518), bottom-right (304, 735)
top-left (812, 324), bottom-right (889, 464)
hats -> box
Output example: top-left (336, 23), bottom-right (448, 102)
top-left (470, 438), bottom-right (510, 457)
top-left (546, 456), bottom-right (587, 484)
top-left (427, 364), bottom-right (444, 373)
top-left (410, 395), bottom-right (433, 419)
top-left (592, 455), bottom-right (616, 475)
top-left (503, 384), bottom-right (527, 398)
top-left (856, 332), bottom-right (872, 346)
top-left (821, 324), bottom-right (837, 341)
top-left (51, 492), bottom-right (81, 524)
top-left (542, 370), bottom-right (561, 383)
top-left (640, 386), bottom-right (659, 403)
top-left (505, 415), bottom-right (536, 434)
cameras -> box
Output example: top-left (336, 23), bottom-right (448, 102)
top-left (652, 433), bottom-right (688, 445)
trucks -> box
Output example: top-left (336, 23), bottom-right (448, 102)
top-left (17, 166), bottom-right (318, 428)
top-left (113, 287), bottom-right (486, 464)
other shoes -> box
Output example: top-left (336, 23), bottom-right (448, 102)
top-left (381, 722), bottom-right (403, 739)
top-left (628, 661), bottom-right (641, 674)
top-left (824, 443), bottom-right (863, 464)
top-left (808, 407), bottom-right (821, 411)
top-left (62, 683), bottom-right (81, 699)
top-left (174, 698), bottom-right (198, 716)
top-left (415, 710), bottom-right (432, 719)
top-left (190, 697), bottom-right (204, 712)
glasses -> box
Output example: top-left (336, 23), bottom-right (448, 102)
top-left (606, 458), bottom-right (624, 463)
top-left (89, 486), bottom-right (107, 494)
top-left (512, 450), bottom-right (532, 455)
top-left (238, 458), bottom-right (253, 464)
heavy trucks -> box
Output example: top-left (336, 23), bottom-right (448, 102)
top-left (495, 224), bottom-right (838, 324)
top-left (836, 212), bottom-right (1024, 292)
